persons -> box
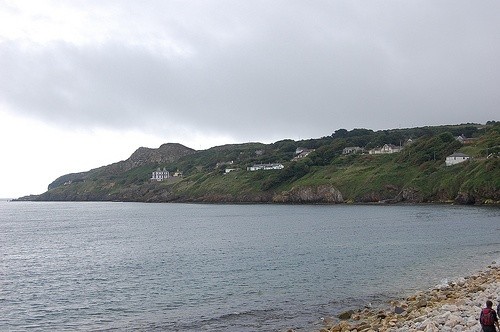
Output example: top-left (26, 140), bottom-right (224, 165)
top-left (479, 300), bottom-right (500, 332)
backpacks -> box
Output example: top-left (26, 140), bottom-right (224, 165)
top-left (481, 309), bottom-right (495, 325)
